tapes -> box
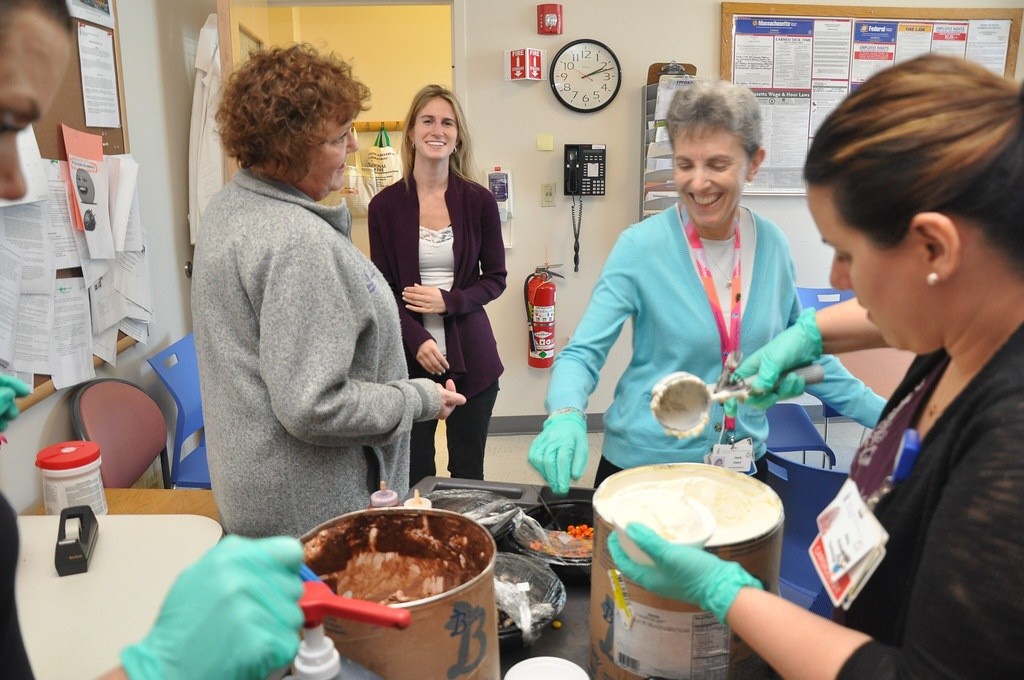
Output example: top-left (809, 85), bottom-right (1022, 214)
top-left (64, 518), bottom-right (82, 540)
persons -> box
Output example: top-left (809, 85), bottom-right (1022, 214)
top-left (367, 85), bottom-right (510, 488)
top-left (610, 54), bottom-right (1022, 680)
top-left (1, 1), bottom-right (303, 679)
top-left (525, 81), bottom-right (887, 496)
top-left (190, 41), bottom-right (468, 537)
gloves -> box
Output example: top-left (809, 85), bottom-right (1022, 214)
top-left (121, 535), bottom-right (307, 680)
top-left (607, 524), bottom-right (764, 628)
top-left (724, 306), bottom-right (824, 417)
top-left (528, 411), bottom-right (589, 496)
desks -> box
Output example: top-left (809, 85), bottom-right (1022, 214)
top-left (14, 514), bottom-right (225, 680)
top-left (20, 488), bottom-right (220, 522)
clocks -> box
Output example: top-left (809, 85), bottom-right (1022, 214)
top-left (550, 39), bottom-right (622, 113)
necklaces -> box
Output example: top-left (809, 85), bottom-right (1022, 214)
top-left (701, 236), bottom-right (735, 290)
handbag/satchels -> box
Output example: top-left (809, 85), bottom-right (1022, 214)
top-left (368, 127), bottom-right (402, 188)
top-left (317, 127), bottom-right (376, 218)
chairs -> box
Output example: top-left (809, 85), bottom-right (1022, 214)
top-left (147, 332), bottom-right (211, 489)
top-left (767, 286), bottom-right (866, 600)
top-left (70, 378), bottom-right (171, 489)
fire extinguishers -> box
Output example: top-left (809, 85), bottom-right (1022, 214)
top-left (523, 264), bottom-right (565, 368)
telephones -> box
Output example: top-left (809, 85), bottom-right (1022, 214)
top-left (564, 143), bottom-right (606, 195)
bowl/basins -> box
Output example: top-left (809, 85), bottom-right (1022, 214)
top-left (505, 499), bottom-right (593, 578)
top-left (409, 489), bottom-right (520, 542)
top-left (612, 492), bottom-right (716, 567)
top-left (492, 552), bottom-right (566, 639)
